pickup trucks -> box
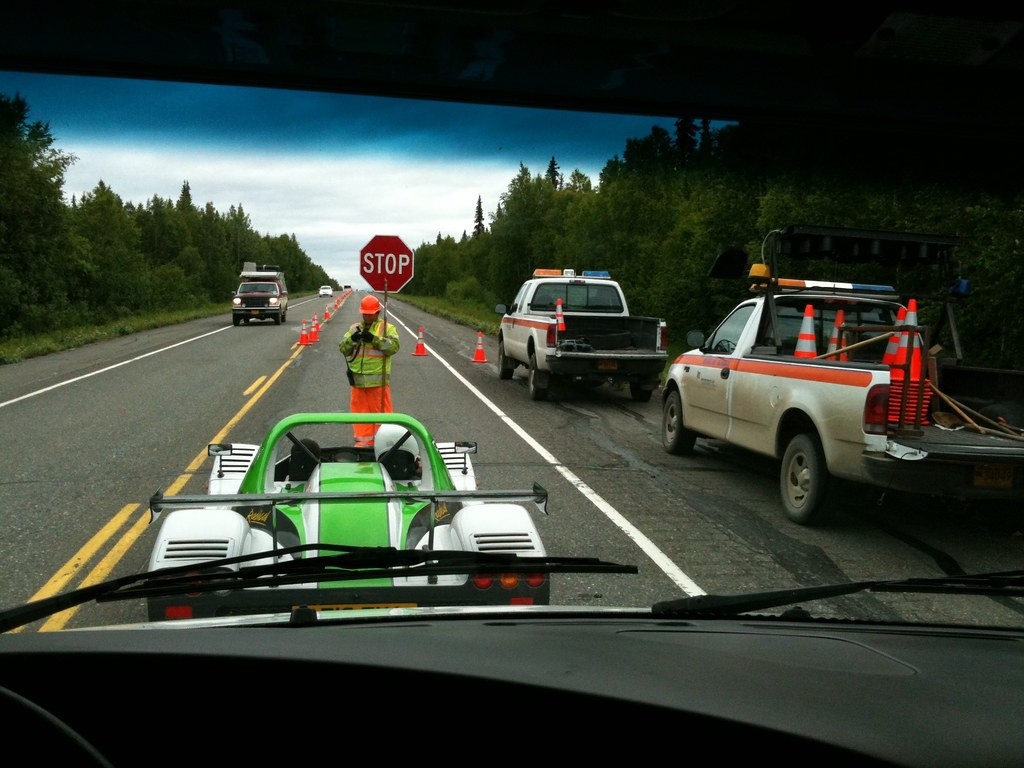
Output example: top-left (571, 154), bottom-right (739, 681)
top-left (231, 265), bottom-right (288, 326)
top-left (492, 268), bottom-right (668, 401)
top-left (654, 261), bottom-right (1020, 525)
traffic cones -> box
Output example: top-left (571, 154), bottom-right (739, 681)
top-left (826, 309), bottom-right (848, 363)
top-left (792, 304), bottom-right (820, 359)
top-left (308, 317), bottom-right (320, 341)
top-left (878, 298), bottom-right (934, 426)
top-left (334, 289), bottom-right (352, 309)
top-left (470, 331), bottom-right (488, 362)
top-left (322, 304), bottom-right (331, 319)
top-left (411, 326), bottom-right (428, 356)
top-left (313, 312), bottom-right (323, 332)
top-left (297, 319), bottom-right (313, 346)
top-left (554, 298), bottom-right (566, 332)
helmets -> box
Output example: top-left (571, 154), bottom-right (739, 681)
top-left (359, 295), bottom-right (381, 314)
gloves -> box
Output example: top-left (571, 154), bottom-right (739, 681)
top-left (351, 330), bottom-right (361, 342)
top-left (361, 330), bottom-right (374, 342)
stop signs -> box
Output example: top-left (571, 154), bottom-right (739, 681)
top-left (360, 235), bottom-right (414, 293)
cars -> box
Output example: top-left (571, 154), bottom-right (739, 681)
top-left (145, 413), bottom-right (548, 621)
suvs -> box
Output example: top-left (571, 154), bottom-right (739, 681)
top-left (318, 285), bottom-right (333, 298)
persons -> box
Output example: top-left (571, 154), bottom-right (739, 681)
top-left (335, 297), bottom-right (403, 444)
